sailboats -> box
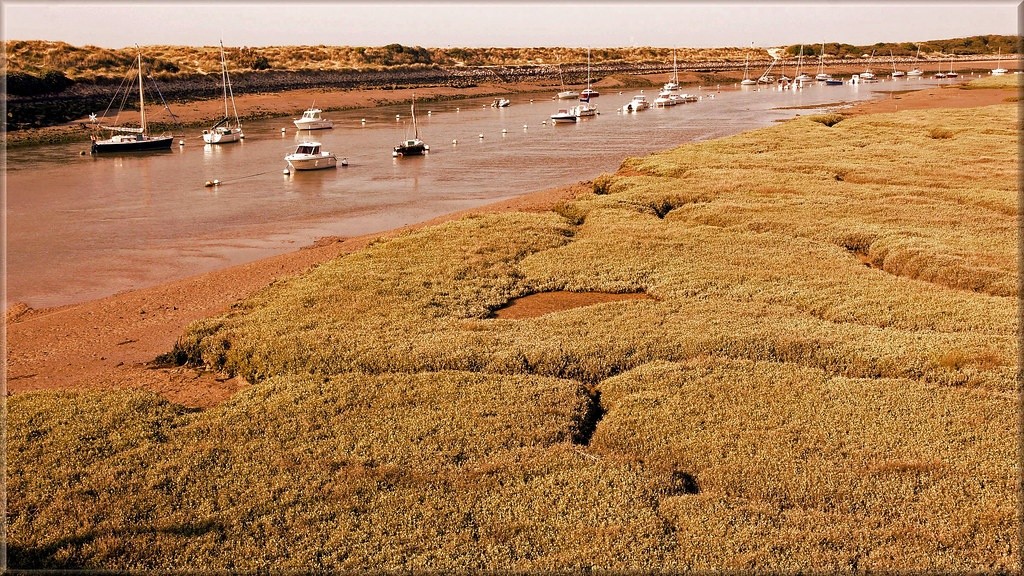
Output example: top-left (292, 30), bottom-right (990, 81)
top-left (778, 45), bottom-right (812, 83)
top-left (90, 43), bottom-right (185, 151)
top-left (393, 94), bottom-right (426, 156)
top-left (992, 47), bottom-right (1009, 74)
top-left (890, 43), bottom-right (957, 78)
top-left (664, 47), bottom-right (679, 90)
top-left (815, 40), bottom-right (831, 81)
top-left (202, 38), bottom-right (244, 144)
top-left (557, 45), bottom-right (599, 98)
top-left (860, 50), bottom-right (876, 80)
top-left (742, 52), bottom-right (777, 84)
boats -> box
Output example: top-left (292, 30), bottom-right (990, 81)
top-left (551, 108), bottom-right (577, 123)
top-left (623, 96), bottom-right (650, 111)
top-left (294, 100), bottom-right (333, 130)
top-left (284, 141), bottom-right (337, 171)
top-left (849, 74), bottom-right (863, 84)
top-left (654, 91), bottom-right (698, 106)
top-left (576, 105), bottom-right (598, 116)
top-left (825, 75), bottom-right (843, 85)
top-left (491, 97), bottom-right (509, 107)
